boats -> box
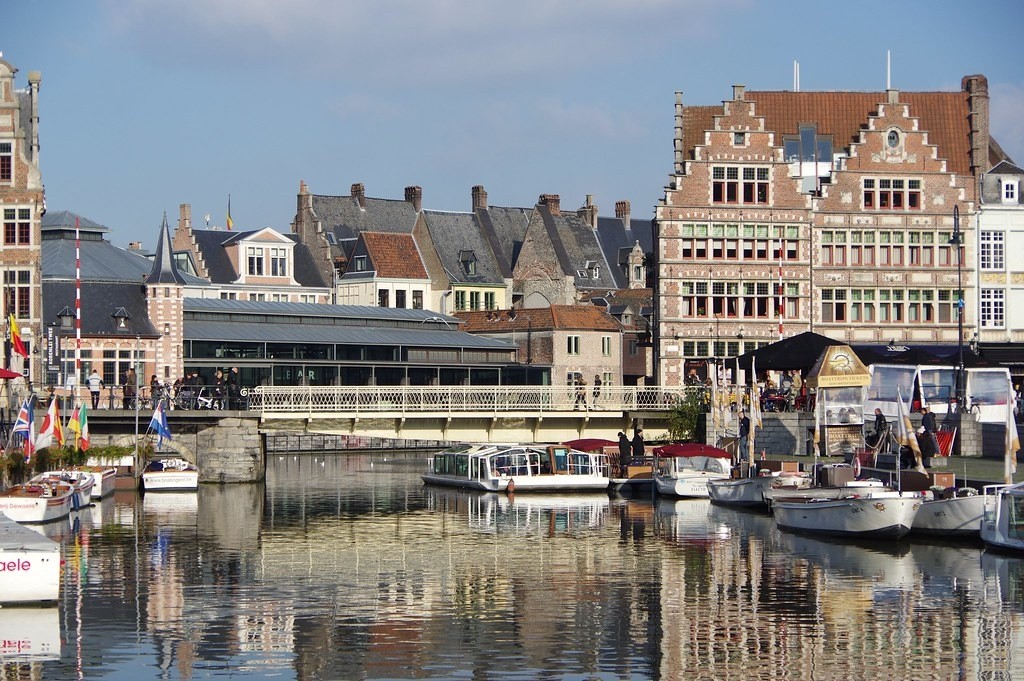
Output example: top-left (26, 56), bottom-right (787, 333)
top-left (0, 467), bottom-right (117, 523)
top-left (421, 442), bottom-right (611, 492)
top-left (651, 443), bottom-right (1024, 556)
top-left (141, 456), bottom-right (201, 490)
top-left (0, 511), bottom-right (60, 605)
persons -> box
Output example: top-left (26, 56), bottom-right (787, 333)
top-left (572, 373), bottom-right (592, 410)
top-left (914, 407), bottom-right (940, 468)
top-left (123, 367), bottom-right (246, 412)
top-left (686, 367), bottom-right (711, 413)
top-left (630, 428), bottom-right (645, 466)
top-left (739, 407), bottom-right (750, 462)
top-left (86, 370), bottom-right (105, 409)
top-left (763, 368), bottom-right (803, 413)
top-left (874, 408), bottom-right (887, 454)
top-left (827, 406), bottom-right (858, 424)
top-left (490, 454), bottom-right (553, 477)
top-left (618, 432), bottom-right (630, 480)
top-left (591, 374), bottom-right (611, 411)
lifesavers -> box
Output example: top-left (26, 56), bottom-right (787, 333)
top-left (853, 457), bottom-right (861, 477)
top-left (780, 472), bottom-right (805, 477)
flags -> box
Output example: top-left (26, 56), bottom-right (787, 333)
top-left (713, 388), bottom-right (742, 432)
top-left (31, 395), bottom-right (65, 453)
top-left (1003, 392), bottom-right (1020, 485)
top-left (227, 196), bottom-right (234, 230)
top-left (148, 399), bottom-right (172, 449)
top-left (148, 527), bottom-right (167, 572)
top-left (9, 308), bottom-right (28, 359)
top-left (67, 403), bottom-right (91, 453)
top-left (753, 381), bottom-right (762, 429)
top-left (896, 388), bottom-right (930, 479)
top-left (813, 392), bottom-right (821, 457)
top-left (14, 397), bottom-right (36, 462)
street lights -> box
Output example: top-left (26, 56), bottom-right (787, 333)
top-left (947, 205), bottom-right (967, 411)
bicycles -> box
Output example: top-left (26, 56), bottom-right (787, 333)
top-left (102, 385), bottom-right (224, 410)
